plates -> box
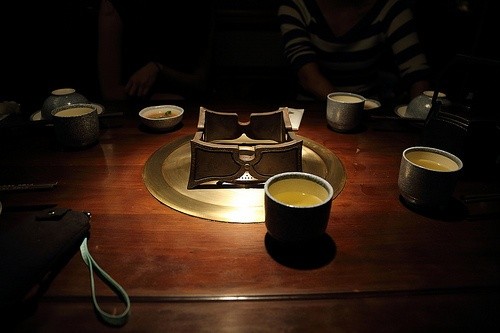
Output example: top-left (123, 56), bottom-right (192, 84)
top-left (30, 104), bottom-right (104, 122)
top-left (394, 105), bottom-right (408, 118)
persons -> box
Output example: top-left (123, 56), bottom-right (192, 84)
top-left (277, 0), bottom-right (432, 102)
top-left (98, 0), bottom-right (213, 101)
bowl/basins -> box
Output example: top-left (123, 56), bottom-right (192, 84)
top-left (404, 91), bottom-right (449, 118)
top-left (138, 105), bottom-right (184, 129)
top-left (41, 88), bottom-right (89, 119)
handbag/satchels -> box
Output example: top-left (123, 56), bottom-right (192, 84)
top-left (420, 87), bottom-right (493, 162)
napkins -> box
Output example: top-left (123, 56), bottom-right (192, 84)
top-left (279, 106), bottom-right (304, 130)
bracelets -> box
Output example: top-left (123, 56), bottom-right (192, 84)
top-left (152, 60), bottom-right (161, 74)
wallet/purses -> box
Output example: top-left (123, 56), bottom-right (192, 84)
top-left (0, 208), bottom-right (130, 327)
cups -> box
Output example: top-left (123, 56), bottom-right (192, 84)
top-left (264, 172), bottom-right (333, 241)
top-left (51, 104), bottom-right (100, 140)
top-left (398, 146), bottom-right (463, 204)
top-left (326, 92), bottom-right (381, 134)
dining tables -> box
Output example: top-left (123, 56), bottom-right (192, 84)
top-left (0, 103), bottom-right (500, 333)
